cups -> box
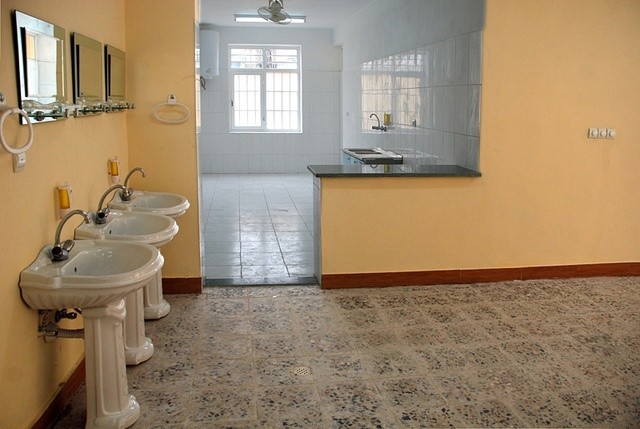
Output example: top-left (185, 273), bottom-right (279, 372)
top-left (384, 114), bottom-right (391, 125)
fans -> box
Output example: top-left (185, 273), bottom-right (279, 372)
top-left (257, 0), bottom-right (292, 24)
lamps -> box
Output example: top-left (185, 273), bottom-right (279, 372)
top-left (232, 13), bottom-right (306, 24)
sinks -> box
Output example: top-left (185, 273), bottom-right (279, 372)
top-left (74, 209), bottom-right (180, 248)
top-left (18, 239), bottom-right (165, 310)
top-left (350, 149), bottom-right (382, 154)
top-left (108, 191), bottom-right (192, 216)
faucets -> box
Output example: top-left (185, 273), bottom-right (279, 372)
top-left (95, 184), bottom-right (131, 224)
top-left (52, 209), bottom-right (92, 260)
top-left (122, 167), bottom-right (146, 200)
top-left (370, 113), bottom-right (387, 132)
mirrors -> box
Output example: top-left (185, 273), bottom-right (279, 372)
top-left (73, 32), bottom-right (109, 118)
top-left (10, 9), bottom-right (67, 125)
top-left (105, 43), bottom-right (136, 112)
